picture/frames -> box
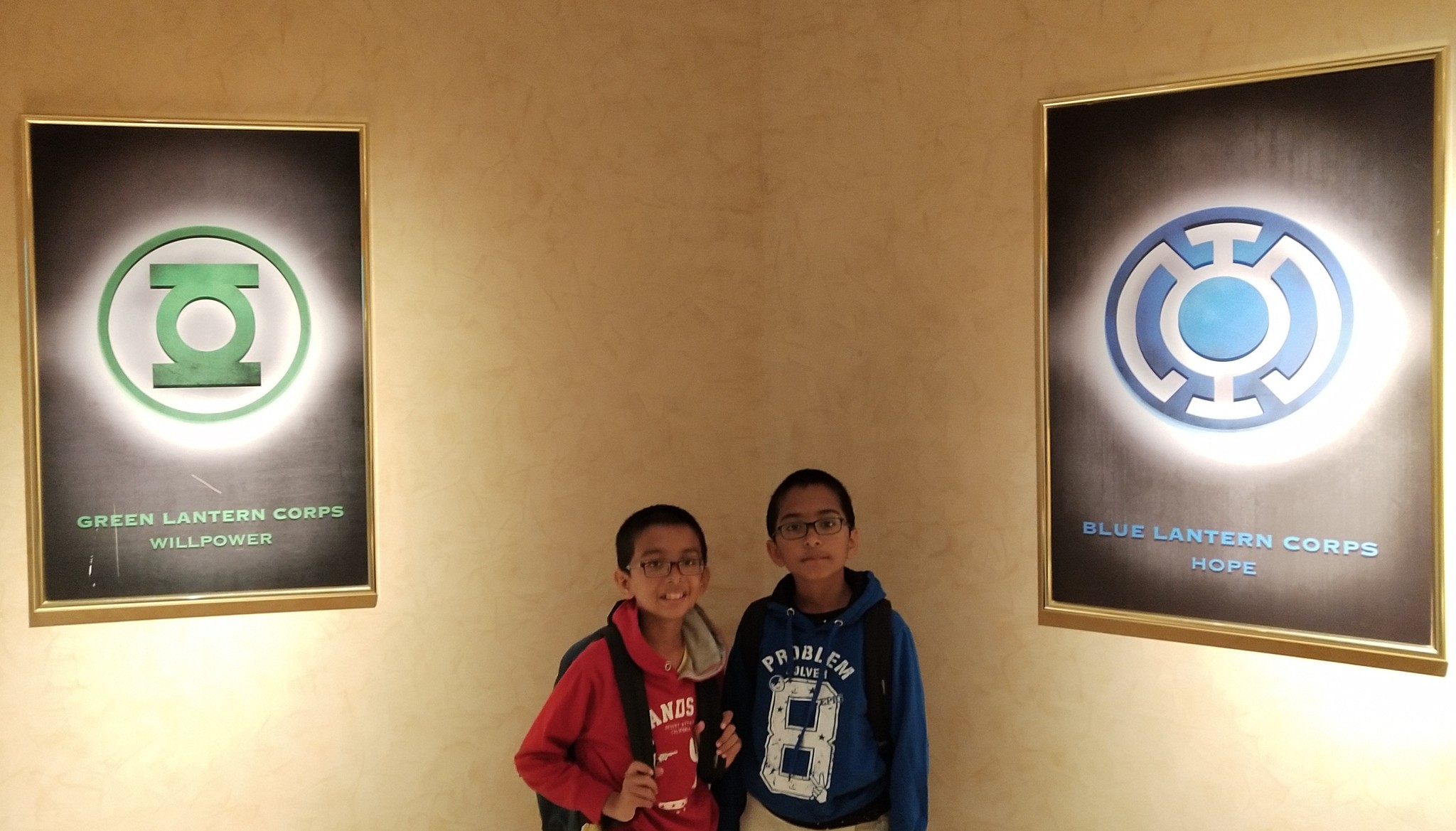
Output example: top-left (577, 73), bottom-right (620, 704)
top-left (1036, 47), bottom-right (1443, 677)
top-left (19, 112), bottom-right (379, 617)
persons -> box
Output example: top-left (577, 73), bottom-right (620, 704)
top-left (512, 504), bottom-right (742, 831)
top-left (722, 468), bottom-right (929, 831)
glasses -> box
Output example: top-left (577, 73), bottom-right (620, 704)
top-left (771, 516), bottom-right (853, 540)
top-left (624, 557), bottom-right (706, 578)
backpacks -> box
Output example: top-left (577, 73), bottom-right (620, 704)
top-left (535, 623), bottom-right (747, 831)
